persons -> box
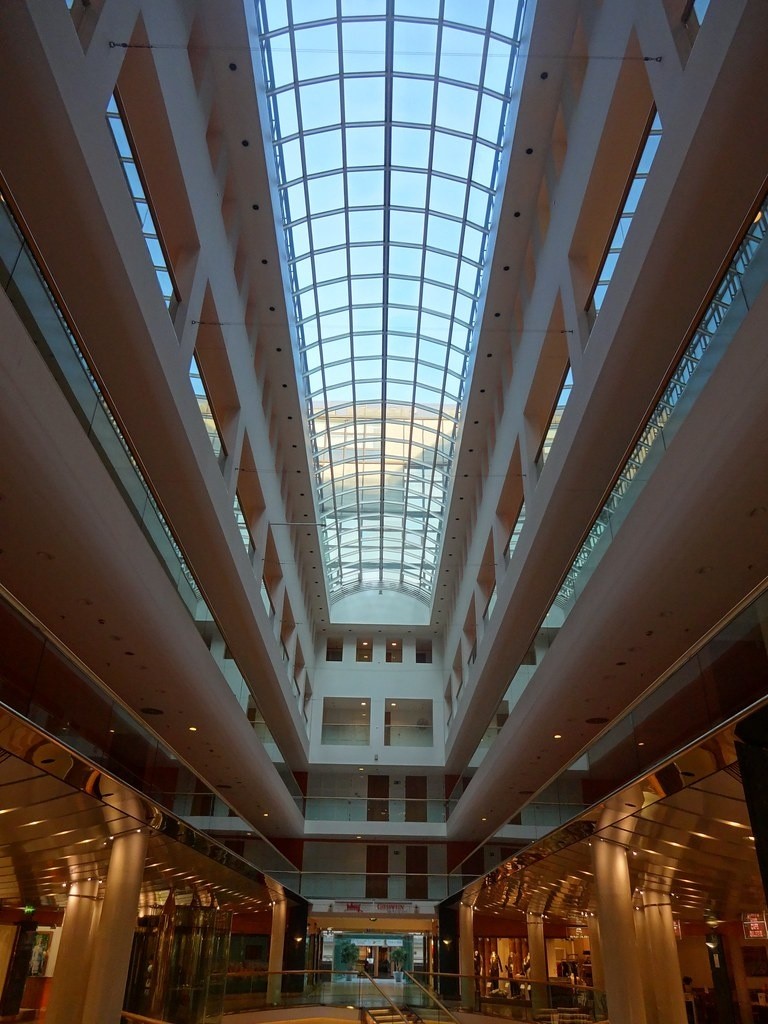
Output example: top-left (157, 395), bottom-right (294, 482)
top-left (474, 950), bottom-right (481, 992)
top-left (507, 953), bottom-right (520, 999)
top-left (31, 939), bottom-right (45, 975)
top-left (489, 951), bottom-right (503, 991)
top-left (524, 954), bottom-right (530, 993)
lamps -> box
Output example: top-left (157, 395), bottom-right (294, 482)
top-left (705, 942), bottom-right (718, 948)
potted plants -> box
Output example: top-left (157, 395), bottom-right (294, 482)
top-left (682, 976), bottom-right (693, 992)
top-left (340, 940), bottom-right (360, 981)
top-left (390, 947), bottom-right (408, 982)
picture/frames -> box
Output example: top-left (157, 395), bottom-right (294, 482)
top-left (26, 931), bottom-right (54, 977)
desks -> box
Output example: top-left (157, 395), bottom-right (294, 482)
top-left (684, 993), bottom-right (698, 1024)
